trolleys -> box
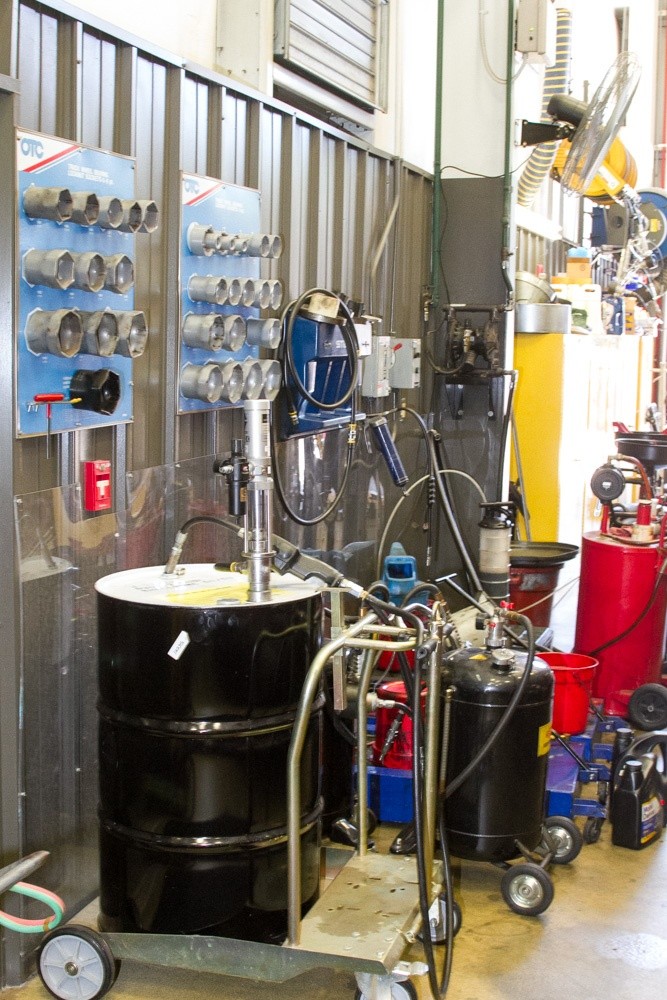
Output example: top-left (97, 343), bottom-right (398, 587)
top-left (354, 697), bottom-right (631, 917)
top-left (31, 606), bottom-right (461, 1000)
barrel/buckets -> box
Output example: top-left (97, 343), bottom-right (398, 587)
top-left (609, 728), bottom-right (667, 826)
top-left (611, 753), bottom-right (667, 849)
top-left (374, 680), bottom-right (427, 770)
top-left (93, 561), bottom-right (327, 950)
top-left (508, 565), bottom-right (564, 628)
top-left (535, 652), bottom-right (599, 736)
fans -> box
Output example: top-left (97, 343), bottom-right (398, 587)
top-left (512, 51), bottom-right (647, 198)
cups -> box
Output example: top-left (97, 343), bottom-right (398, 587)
top-left (566, 246), bottom-right (592, 283)
